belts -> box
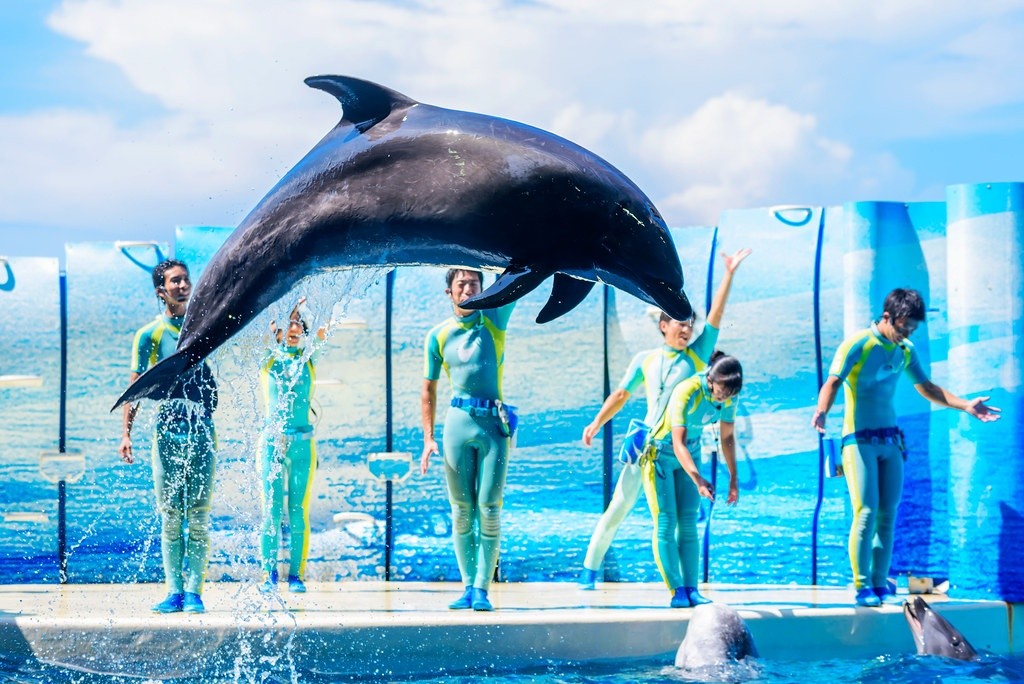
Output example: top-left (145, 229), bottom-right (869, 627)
top-left (841, 427), bottom-right (899, 447)
top-left (451, 397), bottom-right (495, 409)
top-left (663, 437), bottom-right (700, 446)
top-left (288, 432), bottom-right (313, 442)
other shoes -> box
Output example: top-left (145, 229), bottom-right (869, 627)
top-left (855, 588), bottom-right (881, 607)
top-left (471, 588), bottom-right (492, 611)
top-left (151, 593), bottom-right (184, 614)
top-left (875, 587), bottom-right (907, 606)
top-left (578, 567), bottom-right (595, 589)
top-left (183, 591), bottom-right (204, 614)
top-left (259, 570), bottom-right (279, 592)
top-left (671, 587), bottom-right (690, 608)
top-left (687, 587), bottom-right (713, 606)
top-left (288, 575), bottom-right (306, 593)
top-left (449, 585), bottom-right (473, 608)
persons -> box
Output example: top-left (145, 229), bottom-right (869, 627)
top-left (418, 267), bottom-right (516, 610)
top-left (118, 260), bottom-right (213, 616)
top-left (260, 297), bottom-right (333, 593)
top-left (641, 350), bottom-right (740, 610)
top-left (814, 289), bottom-right (1001, 607)
top-left (577, 249), bottom-right (756, 589)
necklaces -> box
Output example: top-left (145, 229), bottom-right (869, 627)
top-left (706, 403), bottom-right (722, 455)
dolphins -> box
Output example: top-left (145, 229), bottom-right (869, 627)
top-left (108, 76), bottom-right (691, 413)
top-left (905, 597), bottom-right (980, 663)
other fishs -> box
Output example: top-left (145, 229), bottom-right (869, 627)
top-left (675, 605), bottom-right (746, 670)
